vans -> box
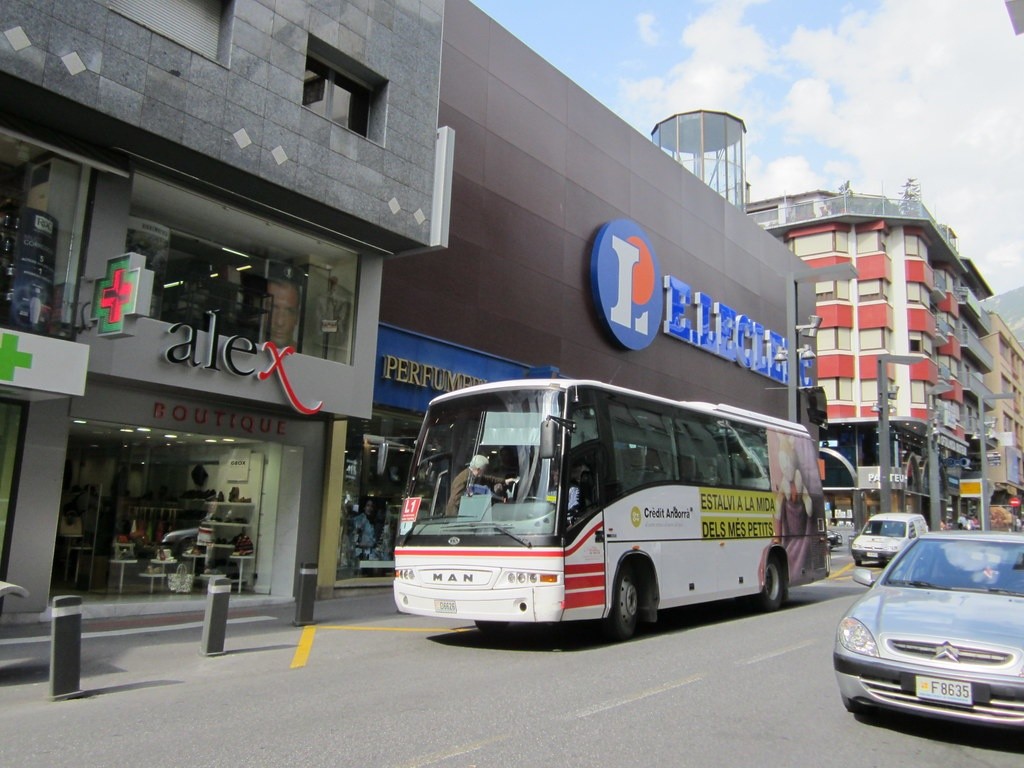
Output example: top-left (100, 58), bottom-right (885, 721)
top-left (851, 512), bottom-right (929, 566)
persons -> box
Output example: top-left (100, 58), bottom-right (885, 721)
top-left (957, 512), bottom-right (980, 531)
top-left (355, 500), bottom-right (378, 547)
top-left (445, 455), bottom-right (517, 516)
top-left (266, 282), bottom-right (299, 352)
top-left (550, 460), bottom-right (598, 507)
top-left (489, 447), bottom-right (520, 478)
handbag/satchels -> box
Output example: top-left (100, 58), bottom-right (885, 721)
top-left (116, 504), bottom-right (179, 543)
top-left (59, 512), bottom-right (82, 535)
top-left (167, 564), bottom-right (195, 594)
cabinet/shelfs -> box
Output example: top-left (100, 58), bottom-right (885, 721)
top-left (196, 500), bottom-right (256, 550)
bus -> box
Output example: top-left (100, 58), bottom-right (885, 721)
top-left (391, 378), bottom-right (829, 641)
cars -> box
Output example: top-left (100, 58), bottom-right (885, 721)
top-left (832, 529), bottom-right (1024, 735)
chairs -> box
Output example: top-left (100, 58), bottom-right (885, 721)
top-left (932, 551), bottom-right (972, 588)
top-left (678, 454), bottom-right (696, 483)
top-left (699, 457), bottom-right (717, 484)
top-left (619, 447), bottom-right (645, 492)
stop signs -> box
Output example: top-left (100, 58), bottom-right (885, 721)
top-left (1008, 496), bottom-right (1021, 507)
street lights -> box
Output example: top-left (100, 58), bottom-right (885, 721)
top-left (921, 379), bottom-right (955, 532)
top-left (870, 353), bottom-right (924, 510)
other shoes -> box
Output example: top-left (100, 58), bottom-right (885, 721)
top-left (179, 487), bottom-right (251, 523)
